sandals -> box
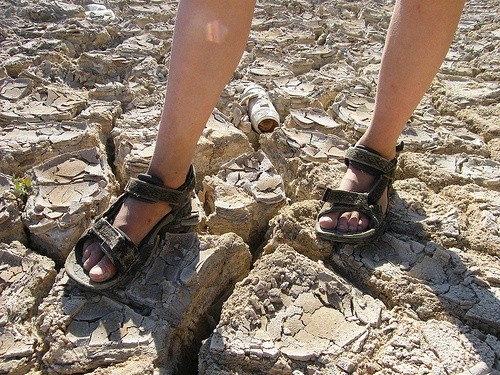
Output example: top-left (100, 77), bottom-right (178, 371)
top-left (64, 163), bottom-right (196, 290)
top-left (313, 143), bottom-right (405, 246)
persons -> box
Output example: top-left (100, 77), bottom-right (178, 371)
top-left (62, 0), bottom-right (469, 293)
